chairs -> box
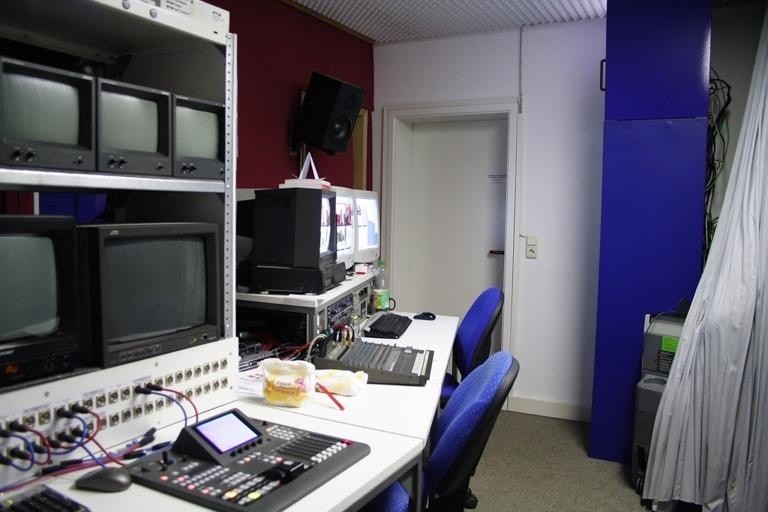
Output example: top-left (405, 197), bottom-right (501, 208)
top-left (440, 286), bottom-right (505, 412)
top-left (367, 351), bottom-right (520, 510)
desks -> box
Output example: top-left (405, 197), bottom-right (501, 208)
top-left (243, 304), bottom-right (461, 440)
top-left (1, 402), bottom-right (427, 512)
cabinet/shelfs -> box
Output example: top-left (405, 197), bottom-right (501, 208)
top-left (0, 0), bottom-right (238, 397)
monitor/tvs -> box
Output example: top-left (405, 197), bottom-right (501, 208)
top-left (0, 214), bottom-right (78, 386)
top-left (236, 187), bottom-right (336, 269)
top-left (77, 223), bottom-right (224, 362)
top-left (353, 189), bottom-right (380, 263)
top-left (330, 185), bottom-right (356, 269)
top-left (0, 54), bottom-right (225, 182)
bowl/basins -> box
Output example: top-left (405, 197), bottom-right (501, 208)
top-left (260, 358), bottom-right (316, 405)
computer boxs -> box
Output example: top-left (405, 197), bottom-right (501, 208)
top-left (247, 263), bottom-right (346, 293)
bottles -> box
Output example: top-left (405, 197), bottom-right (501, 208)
top-left (373, 260), bottom-right (391, 313)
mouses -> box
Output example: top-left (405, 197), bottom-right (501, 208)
top-left (413, 311), bottom-right (435, 320)
top-left (76, 469), bottom-right (132, 492)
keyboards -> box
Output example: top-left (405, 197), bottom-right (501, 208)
top-left (364, 311), bottom-right (411, 339)
top-left (0, 483), bottom-right (88, 512)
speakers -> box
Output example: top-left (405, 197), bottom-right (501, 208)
top-left (301, 70), bottom-right (365, 154)
